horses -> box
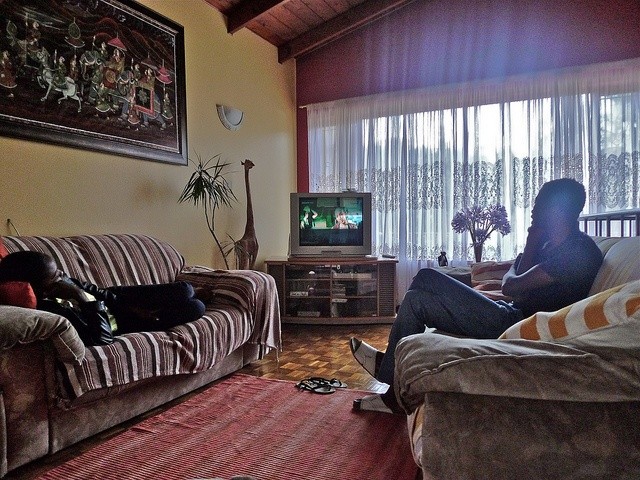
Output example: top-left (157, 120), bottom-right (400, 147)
top-left (37, 50), bottom-right (81, 112)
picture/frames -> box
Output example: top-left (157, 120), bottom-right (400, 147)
top-left (0, 1), bottom-right (187, 166)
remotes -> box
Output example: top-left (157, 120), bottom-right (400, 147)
top-left (382, 254), bottom-right (395, 259)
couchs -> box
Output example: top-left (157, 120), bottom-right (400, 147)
top-left (0, 233), bottom-right (281, 477)
top-left (394, 237), bottom-right (640, 480)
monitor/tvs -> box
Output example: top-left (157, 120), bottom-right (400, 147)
top-left (290, 192), bottom-right (372, 257)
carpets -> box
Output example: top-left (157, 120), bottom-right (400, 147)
top-left (33, 374), bottom-right (416, 480)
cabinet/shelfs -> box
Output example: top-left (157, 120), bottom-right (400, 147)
top-left (263, 259), bottom-right (395, 326)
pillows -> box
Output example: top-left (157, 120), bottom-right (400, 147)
top-left (498, 278), bottom-right (639, 342)
top-left (0, 240), bottom-right (37, 309)
top-left (472, 262), bottom-right (508, 302)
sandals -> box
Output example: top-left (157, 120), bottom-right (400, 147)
top-left (309, 377), bottom-right (349, 389)
top-left (295, 379), bottom-right (336, 394)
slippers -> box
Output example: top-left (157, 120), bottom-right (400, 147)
top-left (353, 395), bottom-right (406, 416)
top-left (349, 336), bottom-right (388, 384)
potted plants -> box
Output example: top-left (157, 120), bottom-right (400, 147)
top-left (451, 207), bottom-right (511, 264)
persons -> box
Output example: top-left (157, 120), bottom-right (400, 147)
top-left (53, 54), bottom-right (67, 88)
top-left (130, 61), bottom-right (142, 84)
top-left (0, 47), bottom-right (19, 98)
top-left (98, 65), bottom-right (105, 79)
top-left (91, 40), bottom-right (109, 63)
top-left (144, 67), bottom-right (154, 84)
top-left (88, 64), bottom-right (99, 107)
top-left (0, 248), bottom-right (207, 348)
top-left (161, 92), bottom-right (174, 129)
top-left (26, 21), bottom-right (42, 48)
top-left (110, 48), bottom-right (119, 71)
top-left (348, 175), bottom-right (604, 420)
top-left (67, 58), bottom-right (79, 82)
top-left (126, 98), bottom-right (142, 132)
top-left (299, 204), bottom-right (318, 231)
top-left (329, 208), bottom-right (360, 229)
top-left (92, 80), bottom-right (115, 122)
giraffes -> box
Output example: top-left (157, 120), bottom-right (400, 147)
top-left (235, 159), bottom-right (259, 270)
